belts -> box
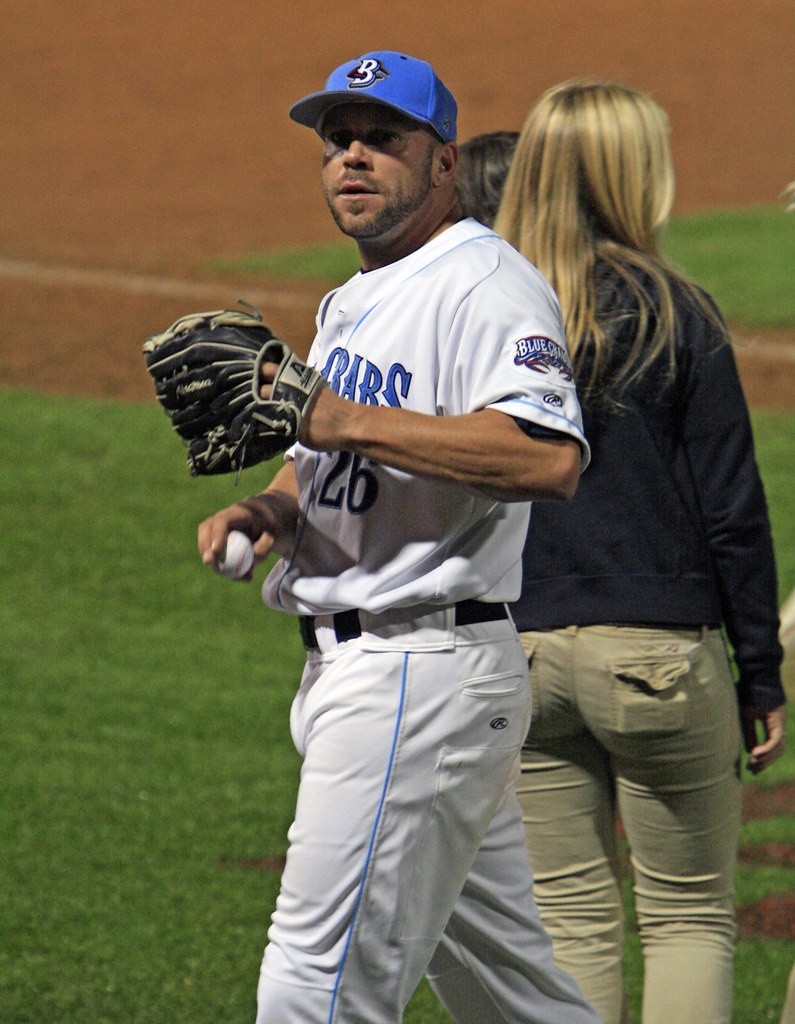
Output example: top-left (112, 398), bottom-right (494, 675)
top-left (299, 592), bottom-right (509, 653)
top-left (598, 620), bottom-right (723, 632)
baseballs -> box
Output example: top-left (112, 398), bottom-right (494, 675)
top-left (209, 528), bottom-right (257, 578)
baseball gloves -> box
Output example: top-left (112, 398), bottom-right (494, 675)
top-left (139, 300), bottom-right (327, 481)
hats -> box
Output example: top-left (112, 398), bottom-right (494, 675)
top-left (289, 51), bottom-right (458, 142)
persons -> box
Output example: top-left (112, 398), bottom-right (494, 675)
top-left (456, 81), bottom-right (787, 1024)
top-left (197, 49), bottom-right (600, 1024)
